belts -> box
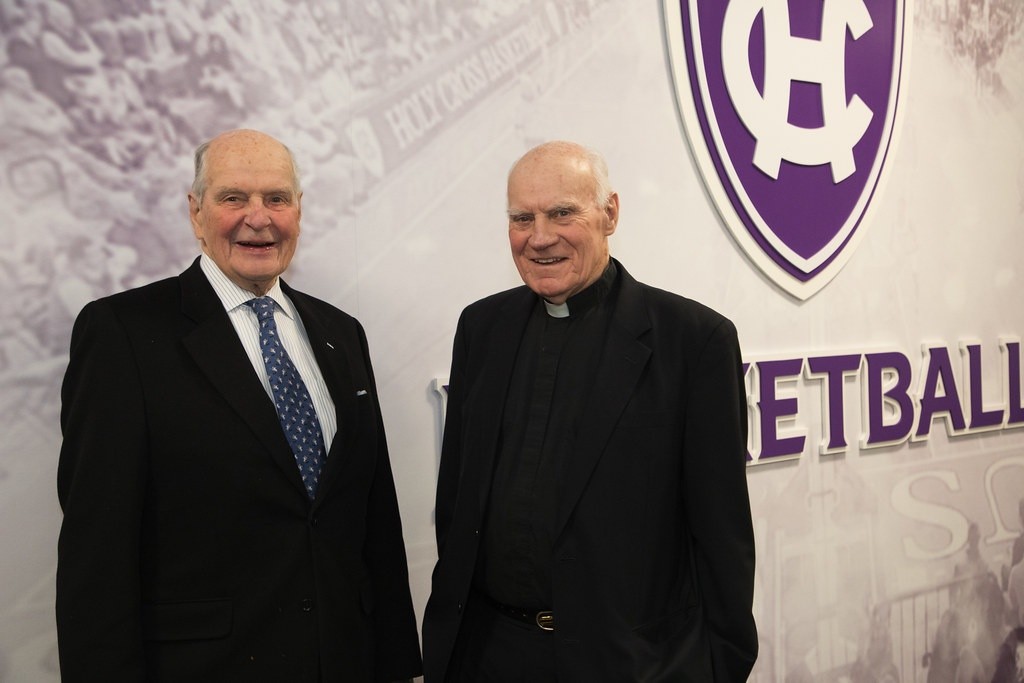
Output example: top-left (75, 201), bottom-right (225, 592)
top-left (486, 597), bottom-right (554, 631)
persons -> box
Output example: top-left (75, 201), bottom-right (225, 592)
top-left (55, 128), bottom-right (423, 683)
top-left (421, 140), bottom-right (760, 683)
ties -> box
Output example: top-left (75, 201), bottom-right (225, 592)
top-left (244, 296), bottom-right (328, 500)
